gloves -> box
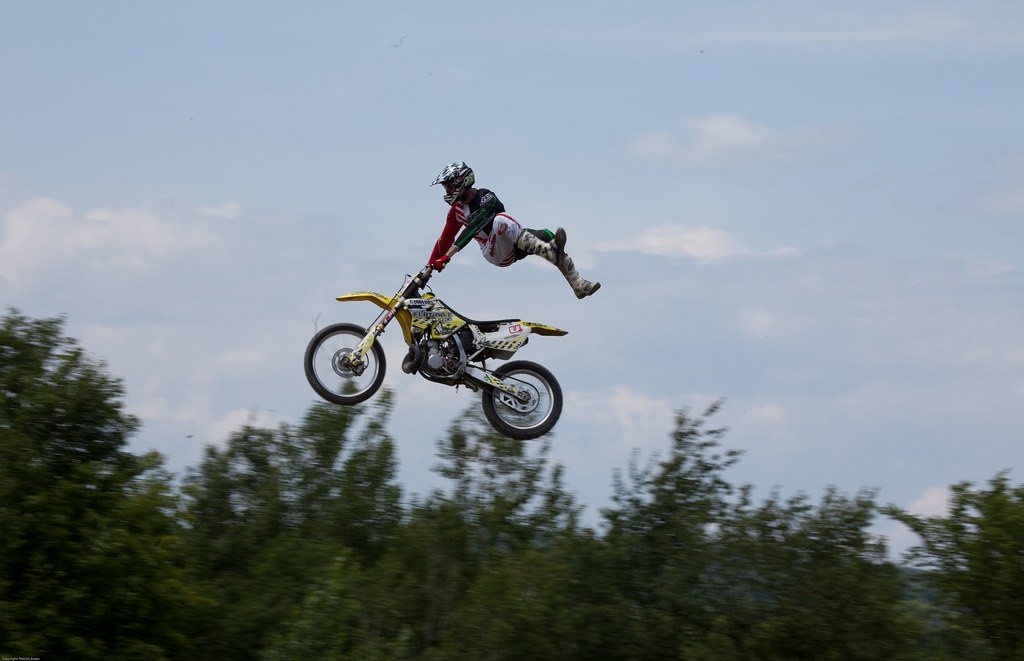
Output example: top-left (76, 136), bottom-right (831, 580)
top-left (434, 254), bottom-right (450, 273)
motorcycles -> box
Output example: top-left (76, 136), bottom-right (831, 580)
top-left (303, 261), bottom-right (570, 441)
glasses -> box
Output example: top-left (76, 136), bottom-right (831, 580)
top-left (442, 181), bottom-right (458, 194)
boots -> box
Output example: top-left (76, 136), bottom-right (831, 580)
top-left (556, 255), bottom-right (601, 299)
top-left (516, 227), bottom-right (566, 266)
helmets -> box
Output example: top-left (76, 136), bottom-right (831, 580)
top-left (430, 162), bottom-right (475, 206)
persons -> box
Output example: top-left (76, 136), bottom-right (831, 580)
top-left (426, 162), bottom-right (601, 299)
